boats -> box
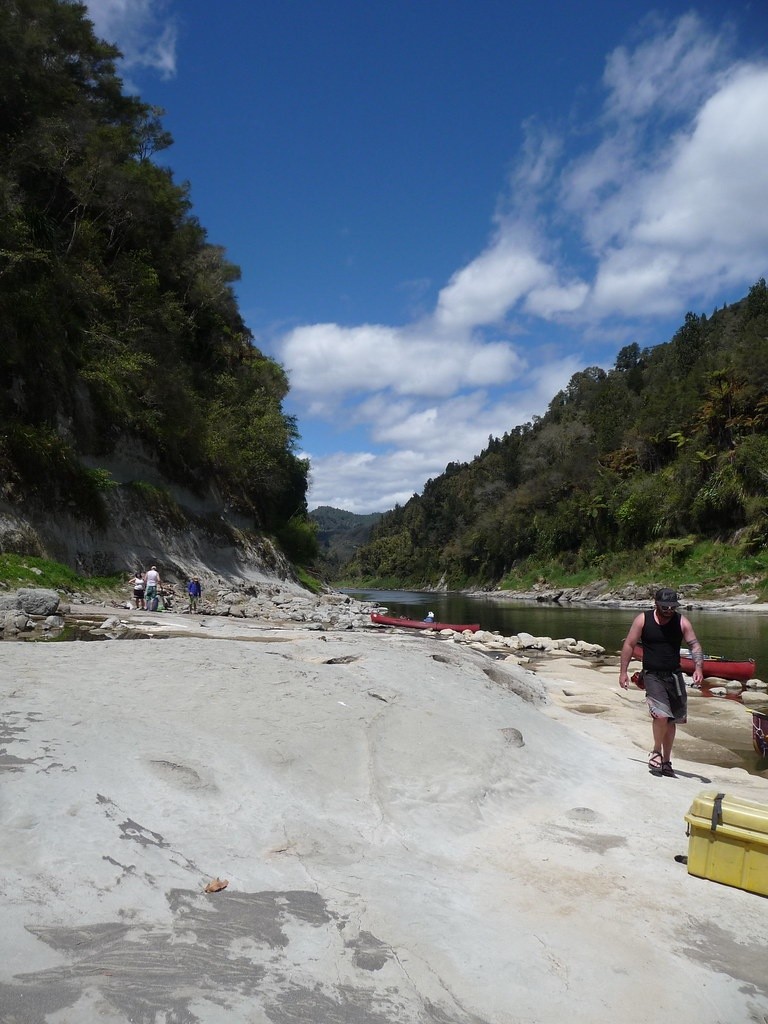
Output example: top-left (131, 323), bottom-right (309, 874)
top-left (633, 648), bottom-right (756, 679)
top-left (371, 611), bottom-right (480, 632)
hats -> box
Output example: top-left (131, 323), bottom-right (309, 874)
top-left (655, 587), bottom-right (679, 607)
top-left (151, 565), bottom-right (157, 570)
top-left (427, 612), bottom-right (434, 618)
top-left (193, 576), bottom-right (198, 582)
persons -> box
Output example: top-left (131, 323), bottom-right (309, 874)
top-left (129, 572), bottom-right (145, 610)
top-left (144, 566), bottom-right (163, 610)
top-left (188, 578), bottom-right (201, 610)
top-left (424, 611), bottom-right (435, 623)
top-left (619, 588), bottom-right (704, 777)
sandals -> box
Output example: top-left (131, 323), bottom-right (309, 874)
top-left (648, 751), bottom-right (674, 776)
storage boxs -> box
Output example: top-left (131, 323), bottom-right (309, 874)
top-left (684, 790), bottom-right (768, 897)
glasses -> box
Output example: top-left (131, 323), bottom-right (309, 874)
top-left (660, 605), bottom-right (677, 611)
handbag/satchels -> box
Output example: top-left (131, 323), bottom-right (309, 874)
top-left (631, 672), bottom-right (645, 690)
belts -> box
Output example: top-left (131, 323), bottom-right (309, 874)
top-left (648, 671), bottom-right (680, 677)
